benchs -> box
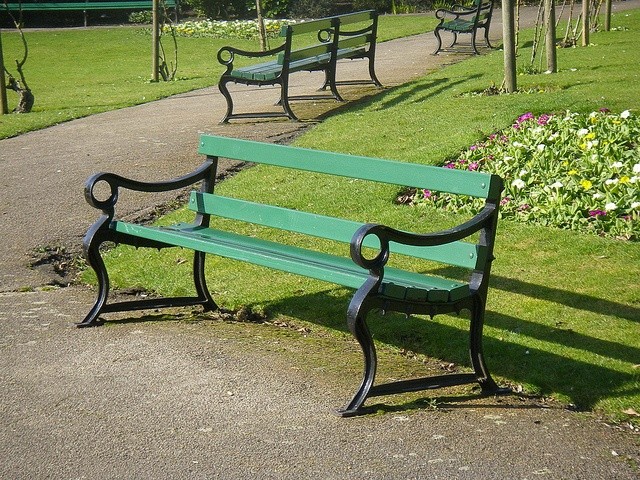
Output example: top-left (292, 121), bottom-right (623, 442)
top-left (431, 0), bottom-right (495, 55)
top-left (0, 0), bottom-right (176, 27)
top-left (218, 9), bottom-right (388, 125)
top-left (75, 134), bottom-right (513, 417)
